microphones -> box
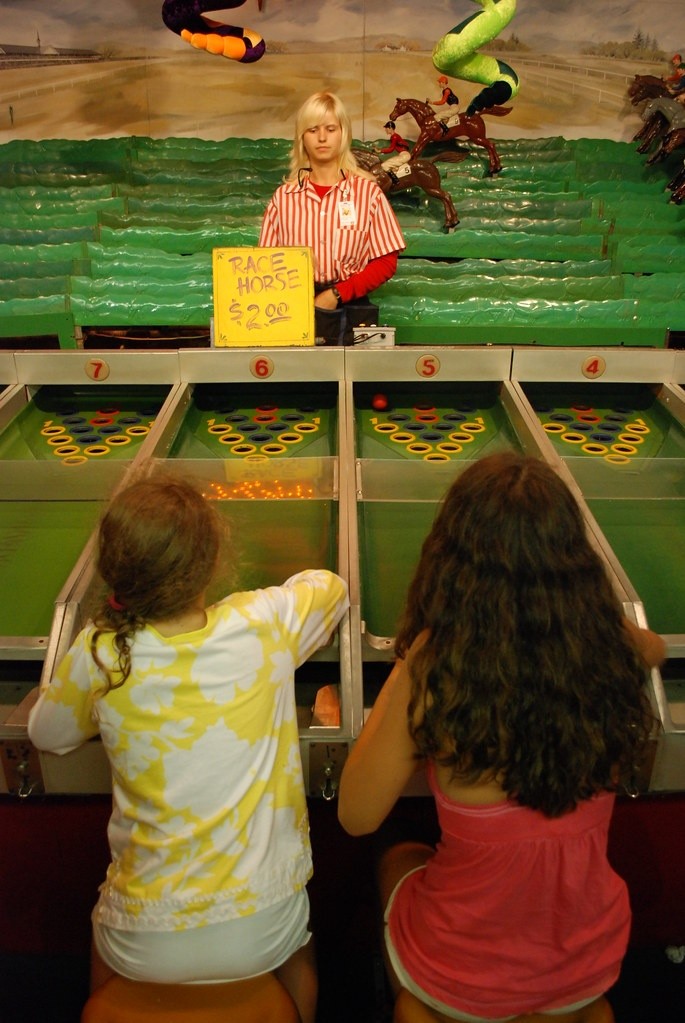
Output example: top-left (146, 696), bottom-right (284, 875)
top-left (301, 167), bottom-right (312, 172)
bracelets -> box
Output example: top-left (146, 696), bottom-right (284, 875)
top-left (332, 287), bottom-right (342, 304)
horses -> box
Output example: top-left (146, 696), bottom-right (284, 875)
top-left (389, 97), bottom-right (514, 174)
top-left (350, 148), bottom-right (470, 229)
top-left (627, 74), bottom-right (685, 206)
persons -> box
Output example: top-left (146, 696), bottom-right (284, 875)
top-left (337, 450), bottom-right (667, 1023)
top-left (257, 92), bottom-right (407, 346)
top-left (666, 55), bottom-right (685, 102)
top-left (372, 121), bottom-right (411, 188)
top-left (427, 75), bottom-right (460, 137)
top-left (27, 477), bottom-right (352, 1023)
